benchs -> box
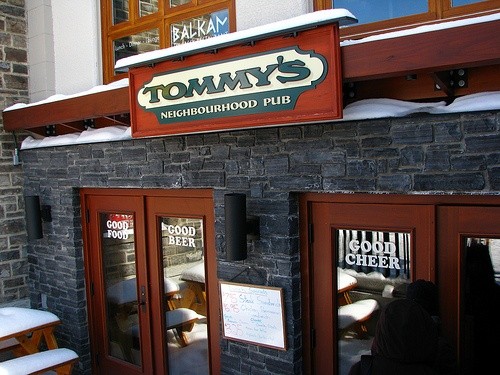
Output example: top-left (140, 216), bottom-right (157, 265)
top-left (127, 318), bottom-right (199, 347)
top-left (339, 310), bottom-right (379, 339)
top-left (28, 357), bottom-right (80, 375)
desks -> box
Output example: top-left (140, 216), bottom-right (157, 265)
top-left (179, 278), bottom-right (207, 316)
top-left (108, 289), bottom-right (179, 322)
top-left (0, 320), bottom-right (61, 355)
top-left (337, 283), bottom-right (359, 304)
